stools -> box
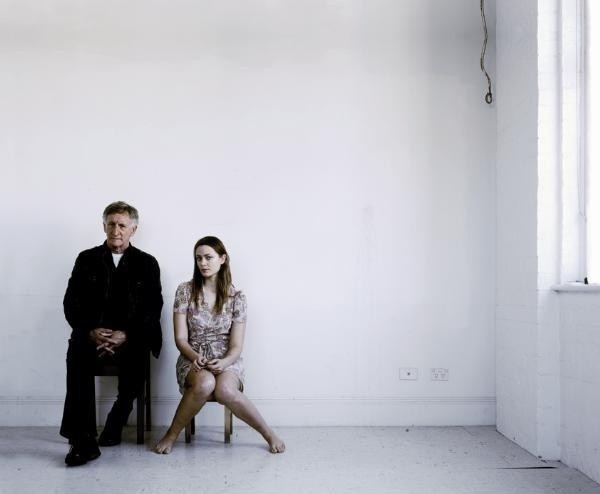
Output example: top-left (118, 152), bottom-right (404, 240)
top-left (92, 353), bottom-right (152, 445)
top-left (185, 387), bottom-right (233, 445)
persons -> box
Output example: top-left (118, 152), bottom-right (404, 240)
top-left (59, 201), bottom-right (163, 466)
top-left (149, 236), bottom-right (285, 455)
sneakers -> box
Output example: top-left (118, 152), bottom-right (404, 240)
top-left (65, 438), bottom-right (101, 465)
top-left (98, 425), bottom-right (124, 447)
